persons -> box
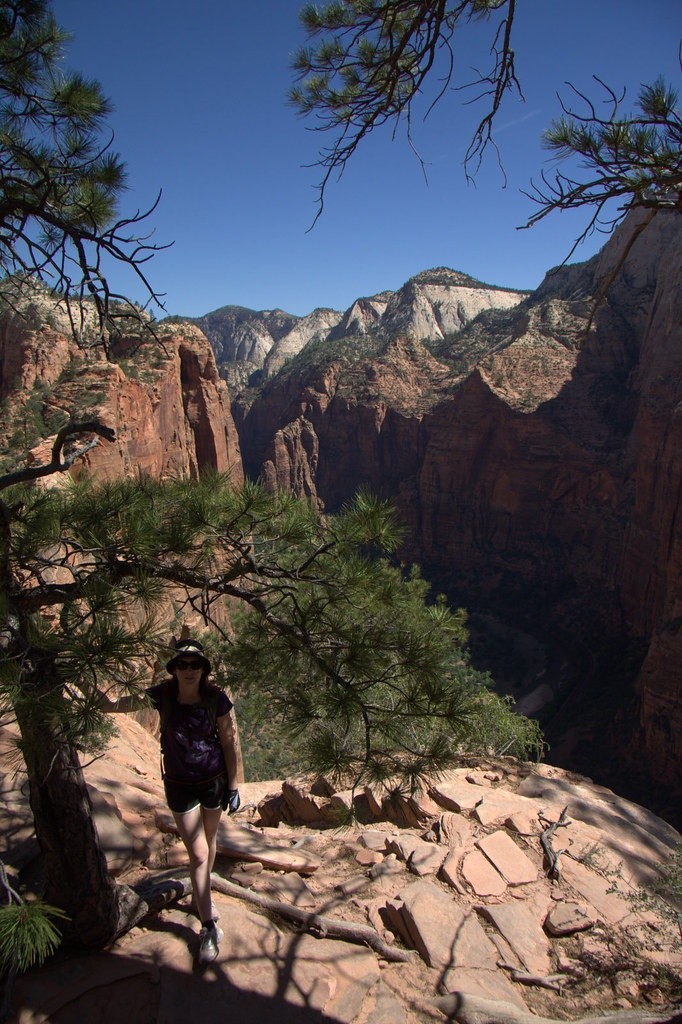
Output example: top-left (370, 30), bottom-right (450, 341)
top-left (68, 640), bottom-right (240, 963)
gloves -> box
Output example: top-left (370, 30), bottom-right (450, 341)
top-left (222, 789), bottom-right (240, 815)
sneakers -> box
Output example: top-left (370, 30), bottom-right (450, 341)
top-left (199, 921), bottom-right (223, 964)
top-left (191, 892), bottom-right (221, 919)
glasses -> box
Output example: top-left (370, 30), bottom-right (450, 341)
top-left (176, 660), bottom-right (203, 670)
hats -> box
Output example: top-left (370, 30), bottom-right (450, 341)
top-left (166, 639), bottom-right (210, 674)
